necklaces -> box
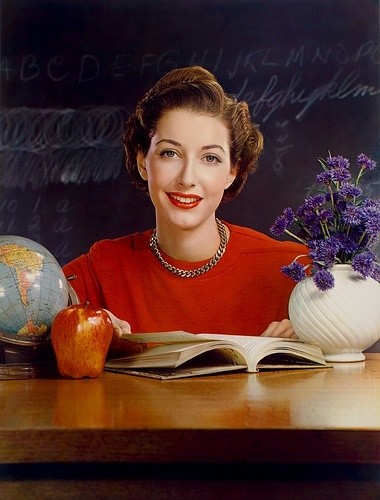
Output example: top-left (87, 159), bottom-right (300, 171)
top-left (148, 219), bottom-right (226, 278)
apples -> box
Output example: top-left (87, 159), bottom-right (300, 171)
top-left (52, 301), bottom-right (114, 379)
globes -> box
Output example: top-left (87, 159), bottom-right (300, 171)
top-left (0, 234), bottom-right (68, 381)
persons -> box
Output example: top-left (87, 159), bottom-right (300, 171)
top-left (59, 66), bottom-right (314, 340)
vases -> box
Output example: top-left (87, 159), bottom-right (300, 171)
top-left (289, 264), bottom-right (380, 361)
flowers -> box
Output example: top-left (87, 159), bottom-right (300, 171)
top-left (270, 150), bottom-right (380, 290)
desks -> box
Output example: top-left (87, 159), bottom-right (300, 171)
top-left (0, 352), bottom-right (380, 500)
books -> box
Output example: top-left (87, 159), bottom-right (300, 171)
top-left (100, 330), bottom-right (335, 382)
